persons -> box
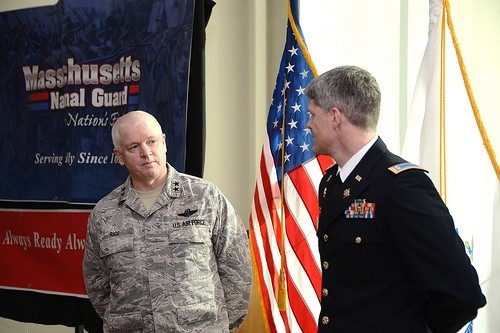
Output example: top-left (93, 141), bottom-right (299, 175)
top-left (302, 65), bottom-right (488, 333)
top-left (80, 110), bottom-right (254, 333)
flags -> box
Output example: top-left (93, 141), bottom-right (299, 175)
top-left (252, 20), bottom-right (340, 333)
